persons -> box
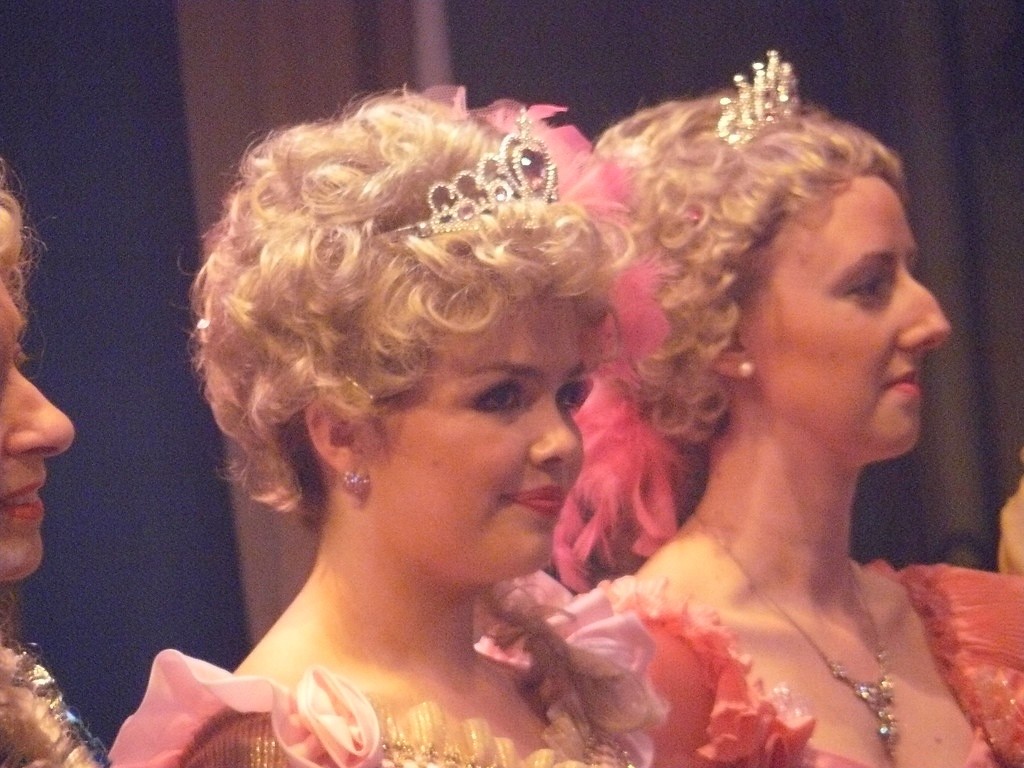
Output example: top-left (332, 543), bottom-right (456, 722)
top-left (475, 51), bottom-right (1024, 768)
top-left (0, 184), bottom-right (111, 768)
top-left (107, 89), bottom-right (816, 768)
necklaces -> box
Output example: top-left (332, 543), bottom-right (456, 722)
top-left (691, 511), bottom-right (900, 768)
